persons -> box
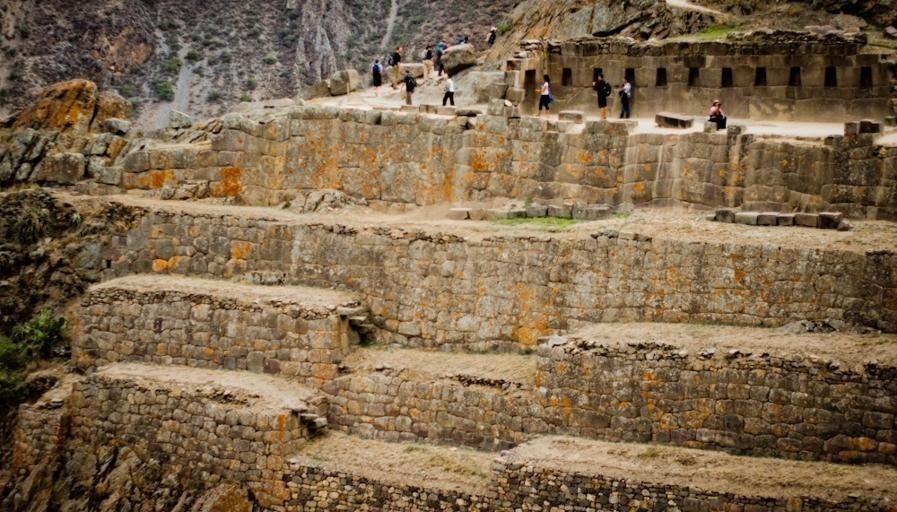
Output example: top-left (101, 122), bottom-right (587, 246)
top-left (403, 70), bottom-right (417, 104)
top-left (371, 46), bottom-right (403, 96)
top-left (421, 35), bottom-right (468, 76)
top-left (534, 74), bottom-right (550, 116)
top-left (487, 28), bottom-right (497, 48)
top-left (709, 99), bottom-right (726, 130)
top-left (592, 72), bottom-right (632, 119)
top-left (442, 74), bottom-right (455, 106)
